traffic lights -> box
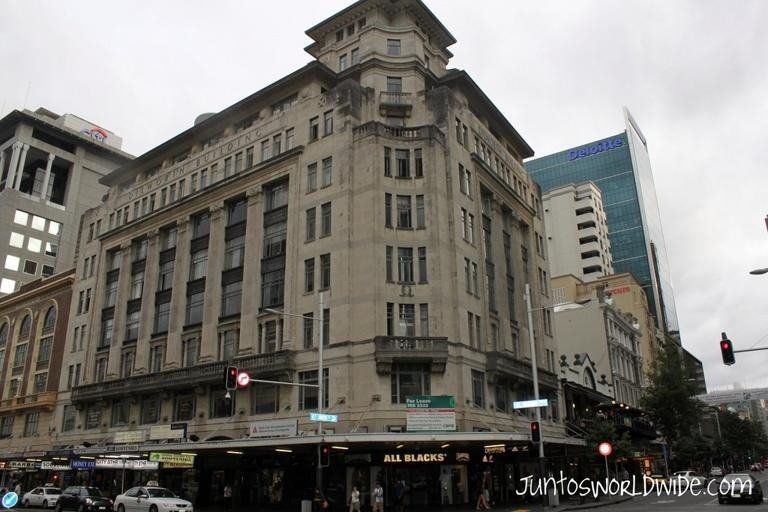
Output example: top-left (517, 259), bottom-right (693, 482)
top-left (226, 365), bottom-right (237, 391)
top-left (319, 443), bottom-right (331, 467)
top-left (720, 339), bottom-right (736, 364)
top-left (530, 421), bottom-right (541, 445)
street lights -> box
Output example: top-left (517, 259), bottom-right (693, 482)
top-left (749, 268), bottom-right (768, 274)
top-left (525, 281), bottom-right (591, 507)
top-left (264, 289), bottom-right (325, 511)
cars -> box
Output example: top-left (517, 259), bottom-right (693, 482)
top-left (718, 473), bottom-right (762, 505)
top-left (647, 471), bottom-right (709, 490)
top-left (21, 480), bottom-right (193, 512)
top-left (751, 460), bottom-right (768, 472)
top-left (710, 466), bottom-right (723, 476)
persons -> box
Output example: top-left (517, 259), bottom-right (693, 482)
top-left (371, 482), bottom-right (384, 512)
top-left (320, 496), bottom-right (330, 512)
top-left (620, 467), bottom-right (631, 491)
top-left (223, 482), bottom-right (233, 508)
top-left (13, 480), bottom-right (23, 505)
top-left (392, 479), bottom-right (405, 511)
top-left (473, 472), bottom-right (492, 510)
top-left (480, 470), bottom-right (490, 507)
top-left (346, 484), bottom-right (361, 512)
top-left (313, 487), bottom-right (321, 512)
